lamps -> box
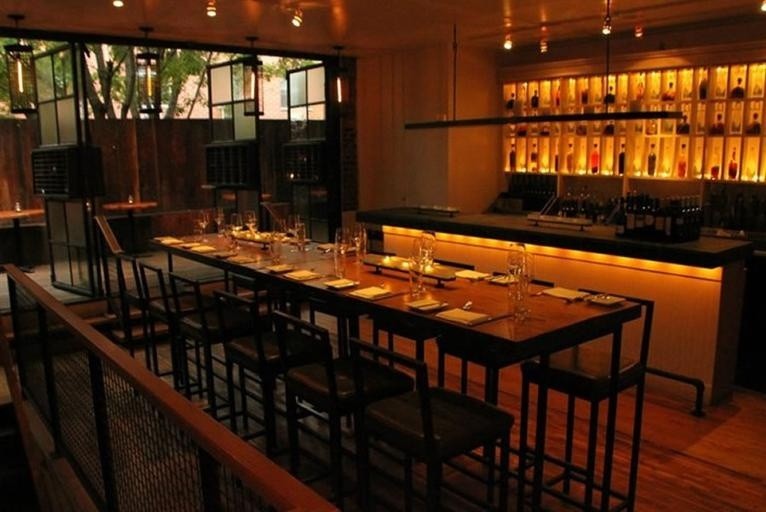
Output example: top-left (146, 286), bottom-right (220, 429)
top-left (7, 13), bottom-right (264, 117)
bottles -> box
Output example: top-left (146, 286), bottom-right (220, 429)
top-left (503, 85), bottom-right (616, 113)
top-left (636, 78), bottom-right (746, 100)
top-left (590, 141), bottom-right (686, 180)
top-left (507, 141), bottom-right (574, 174)
top-left (621, 113), bottom-right (760, 135)
top-left (559, 184), bottom-right (702, 242)
top-left (508, 121), bottom-right (617, 136)
top-left (709, 144), bottom-right (757, 180)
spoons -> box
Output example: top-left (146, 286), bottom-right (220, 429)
top-left (435, 301), bottom-right (474, 317)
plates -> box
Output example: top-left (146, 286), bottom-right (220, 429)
top-left (407, 298), bottom-right (447, 313)
top-left (266, 263), bottom-right (298, 273)
top-left (584, 294), bottom-right (625, 305)
top-left (324, 278), bottom-right (358, 290)
top-left (485, 273), bottom-right (519, 284)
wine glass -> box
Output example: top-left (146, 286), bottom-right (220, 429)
top-left (292, 223), bottom-right (308, 252)
top-left (197, 210), bottom-right (210, 243)
top-left (408, 259), bottom-right (426, 297)
top-left (271, 217), bottom-right (287, 262)
top-left (351, 224), bottom-right (368, 265)
top-left (213, 207), bottom-right (224, 238)
top-left (334, 227), bottom-right (353, 278)
top-left (229, 213), bottom-right (243, 251)
top-left (244, 210), bottom-right (257, 245)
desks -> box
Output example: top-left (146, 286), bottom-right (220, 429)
top-left (102, 201), bottom-right (156, 255)
top-left (3, 206), bottom-right (45, 273)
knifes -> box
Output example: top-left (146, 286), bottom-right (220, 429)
top-left (467, 312), bottom-right (512, 327)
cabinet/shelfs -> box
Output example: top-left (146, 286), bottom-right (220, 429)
top-left (500, 60), bottom-right (766, 186)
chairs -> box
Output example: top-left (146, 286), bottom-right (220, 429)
top-left (115, 245), bottom-right (654, 510)
